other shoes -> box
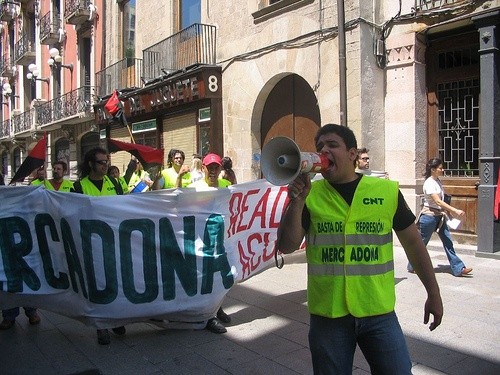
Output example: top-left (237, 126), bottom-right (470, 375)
top-left (112, 325), bottom-right (126, 335)
top-left (29, 313), bottom-right (40, 325)
top-left (97, 329), bottom-right (110, 345)
top-left (408, 268), bottom-right (417, 273)
top-left (0, 318), bottom-right (15, 329)
top-left (452, 266), bottom-right (472, 276)
top-left (206, 317), bottom-right (227, 333)
top-left (216, 307), bottom-right (231, 323)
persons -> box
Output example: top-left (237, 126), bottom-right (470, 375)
top-left (0, 148), bottom-right (236, 344)
top-left (278, 125), bottom-right (444, 375)
top-left (354, 148), bottom-right (389, 180)
top-left (406, 157), bottom-right (472, 277)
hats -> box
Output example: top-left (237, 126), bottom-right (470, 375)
top-left (203, 153), bottom-right (223, 167)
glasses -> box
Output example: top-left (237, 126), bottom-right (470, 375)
top-left (95, 159), bottom-right (107, 165)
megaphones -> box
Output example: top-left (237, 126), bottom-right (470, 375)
top-left (260, 136), bottom-right (330, 200)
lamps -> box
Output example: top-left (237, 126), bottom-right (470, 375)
top-left (47, 48), bottom-right (73, 74)
top-left (2, 83), bottom-right (20, 99)
top-left (27, 63), bottom-right (50, 86)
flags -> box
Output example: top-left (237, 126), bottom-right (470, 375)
top-left (8, 131), bottom-right (47, 184)
top-left (105, 92), bottom-right (128, 127)
top-left (107, 138), bottom-right (163, 171)
